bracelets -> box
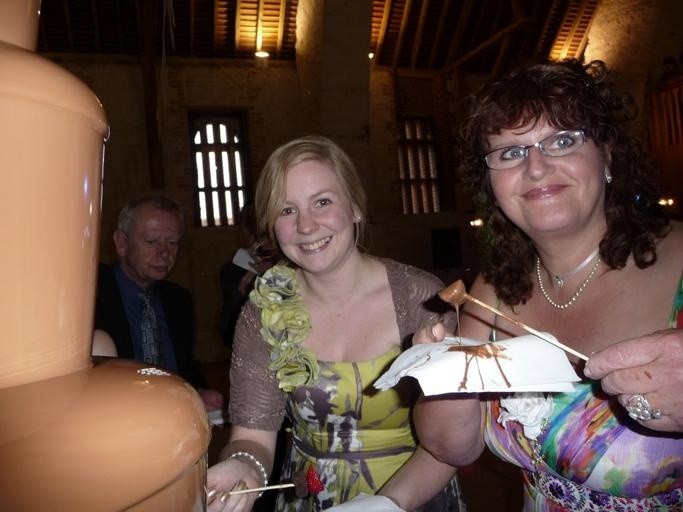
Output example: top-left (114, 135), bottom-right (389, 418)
top-left (223, 450), bottom-right (268, 502)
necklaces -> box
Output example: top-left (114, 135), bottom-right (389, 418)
top-left (534, 251), bottom-right (601, 309)
top-left (539, 244), bottom-right (600, 290)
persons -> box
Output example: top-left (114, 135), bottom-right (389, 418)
top-left (405, 53), bottom-right (680, 509)
top-left (91, 192), bottom-right (227, 433)
top-left (89, 325), bottom-right (119, 359)
top-left (214, 196), bottom-right (285, 350)
top-left (201, 133), bottom-right (472, 511)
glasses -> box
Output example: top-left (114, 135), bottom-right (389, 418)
top-left (477, 126), bottom-right (590, 172)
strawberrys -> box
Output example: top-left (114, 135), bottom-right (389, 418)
top-left (306, 465), bottom-right (324, 493)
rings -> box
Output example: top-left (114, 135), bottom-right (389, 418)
top-left (621, 392), bottom-right (662, 422)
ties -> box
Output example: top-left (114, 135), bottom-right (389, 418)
top-left (135, 292), bottom-right (161, 368)
